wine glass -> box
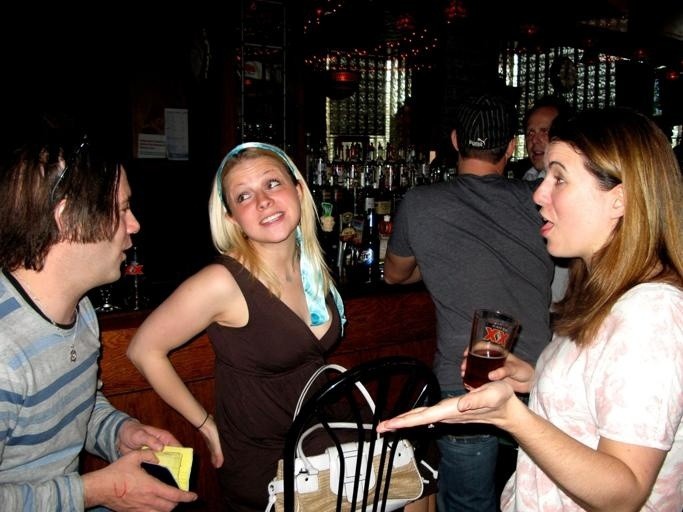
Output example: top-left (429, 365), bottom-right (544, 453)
top-left (92, 282), bottom-right (119, 314)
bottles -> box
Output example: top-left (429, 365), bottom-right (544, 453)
top-left (243, 119), bottom-right (282, 147)
top-left (119, 246), bottom-right (151, 310)
top-left (304, 129), bottom-right (458, 284)
top-left (507, 156), bottom-right (518, 180)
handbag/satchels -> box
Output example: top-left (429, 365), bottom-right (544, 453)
top-left (267, 365), bottom-right (424, 512)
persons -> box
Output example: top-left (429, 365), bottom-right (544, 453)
top-left (126, 141), bottom-right (347, 512)
top-left (522, 97), bottom-right (572, 304)
top-left (383, 90), bottom-right (556, 512)
top-left (0, 133), bottom-right (198, 512)
top-left (377, 106), bottom-right (682, 512)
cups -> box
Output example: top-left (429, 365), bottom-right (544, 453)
top-left (462, 308), bottom-right (520, 393)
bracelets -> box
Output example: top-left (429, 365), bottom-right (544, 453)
top-left (197, 413), bottom-right (209, 430)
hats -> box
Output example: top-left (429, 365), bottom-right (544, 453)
top-left (456, 93), bottom-right (519, 149)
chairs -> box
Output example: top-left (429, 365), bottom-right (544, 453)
top-left (263, 353), bottom-right (441, 510)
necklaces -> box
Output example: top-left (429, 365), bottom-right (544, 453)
top-left (12, 272), bottom-right (80, 362)
